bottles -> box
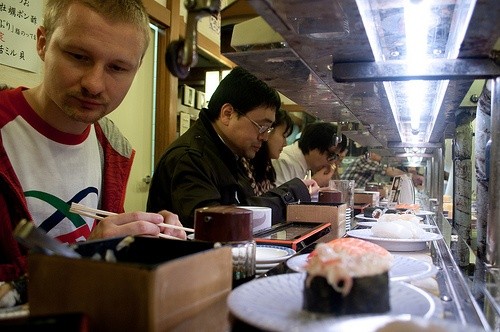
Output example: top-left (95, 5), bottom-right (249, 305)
top-left (194, 206), bottom-right (256, 289)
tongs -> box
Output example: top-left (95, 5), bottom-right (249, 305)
top-left (11, 219), bottom-right (81, 258)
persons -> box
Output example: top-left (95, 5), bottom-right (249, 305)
top-left (0, 0), bottom-right (186, 283)
top-left (342, 134), bottom-right (423, 190)
top-left (146, 66), bottom-right (320, 226)
top-left (241, 108), bottom-right (348, 197)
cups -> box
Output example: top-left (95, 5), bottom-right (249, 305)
top-left (329, 179), bottom-right (354, 220)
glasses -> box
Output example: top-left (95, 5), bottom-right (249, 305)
top-left (234, 108), bottom-right (274, 136)
top-left (316, 143), bottom-right (340, 163)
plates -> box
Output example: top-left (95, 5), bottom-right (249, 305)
top-left (416, 211), bottom-right (435, 218)
top-left (287, 254), bottom-right (433, 281)
top-left (347, 229), bottom-right (443, 251)
top-left (356, 222), bottom-right (438, 228)
top-left (227, 272), bottom-right (444, 332)
top-left (355, 214), bottom-right (423, 222)
top-left (256, 245), bottom-right (297, 264)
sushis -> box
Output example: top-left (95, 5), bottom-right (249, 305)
top-left (303, 237), bottom-right (396, 314)
